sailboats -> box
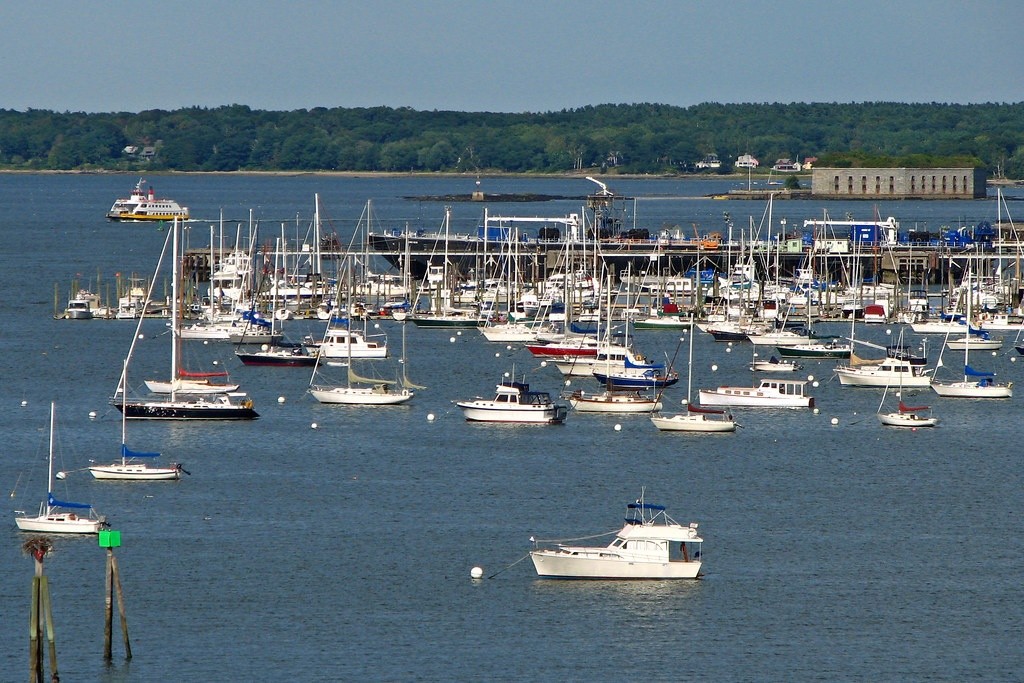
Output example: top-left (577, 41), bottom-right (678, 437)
top-left (877, 327), bottom-right (941, 427)
top-left (566, 279), bottom-right (680, 414)
top-left (10, 402), bottom-right (108, 534)
top-left (307, 255), bottom-right (415, 405)
top-left (648, 314), bottom-right (744, 432)
top-left (87, 360), bottom-right (181, 479)
top-left (832, 234), bottom-right (932, 388)
top-left (177, 162), bottom-right (1024, 366)
top-left (109, 215), bottom-right (261, 420)
top-left (929, 252), bottom-right (1013, 398)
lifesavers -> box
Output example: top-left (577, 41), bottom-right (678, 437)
top-left (635, 354), bottom-right (641, 360)
top-left (69, 513), bottom-right (75, 520)
top-left (402, 390), bottom-right (409, 396)
top-left (245, 401), bottom-right (253, 408)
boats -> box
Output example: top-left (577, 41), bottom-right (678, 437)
top-left (529, 485), bottom-right (703, 580)
top-left (64, 276), bottom-right (101, 319)
top-left (365, 232), bottom-right (1006, 286)
top-left (106, 175), bottom-right (189, 222)
top-left (457, 361), bottom-right (566, 423)
top-left (699, 345), bottom-right (815, 408)
top-left (116, 274), bottom-right (147, 321)
